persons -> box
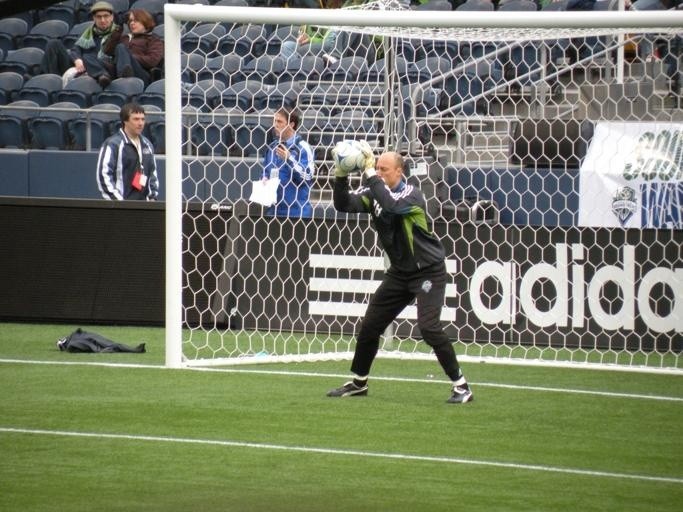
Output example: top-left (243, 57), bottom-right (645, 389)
top-left (325, 139), bottom-right (474, 406)
top-left (34, 2), bottom-right (123, 80)
top-left (279, 23), bottom-right (341, 58)
top-left (563, 0), bottom-right (597, 68)
top-left (82, 7), bottom-right (164, 89)
top-left (653, 0), bottom-right (683, 108)
top-left (95, 101), bottom-right (159, 201)
top-left (258, 104), bottom-right (316, 217)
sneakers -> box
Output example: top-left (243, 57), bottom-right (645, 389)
top-left (324, 380), bottom-right (369, 399)
top-left (445, 387), bottom-right (475, 404)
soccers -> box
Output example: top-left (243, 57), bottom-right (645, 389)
top-left (335, 140), bottom-right (373, 172)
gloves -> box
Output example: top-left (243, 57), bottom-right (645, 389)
top-left (330, 147), bottom-right (351, 178)
top-left (357, 137), bottom-right (377, 174)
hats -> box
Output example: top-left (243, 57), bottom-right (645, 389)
top-left (89, 0), bottom-right (115, 15)
